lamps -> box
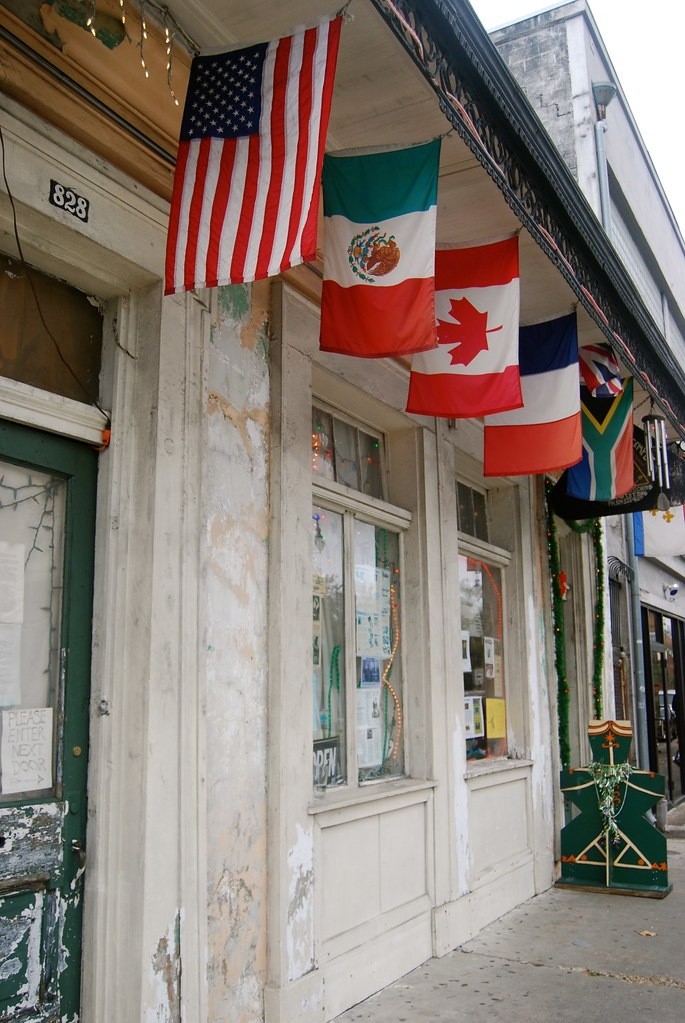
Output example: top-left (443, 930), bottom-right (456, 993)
top-left (662, 582), bottom-right (679, 602)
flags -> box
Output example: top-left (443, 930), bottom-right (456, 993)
top-left (318, 134), bottom-right (451, 360)
top-left (564, 375), bottom-right (636, 501)
top-left (163, 13), bottom-right (345, 296)
top-left (481, 303), bottom-right (585, 478)
top-left (633, 505), bottom-right (685, 557)
top-left (404, 229), bottom-right (527, 418)
